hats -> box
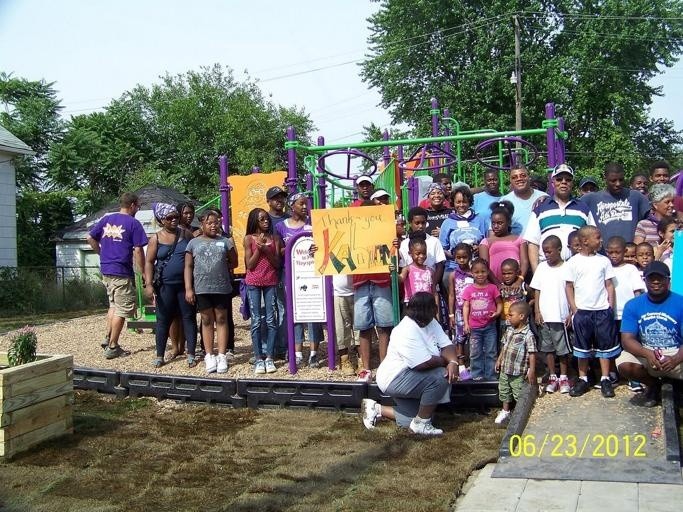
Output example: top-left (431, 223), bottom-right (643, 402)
top-left (266, 186), bottom-right (288, 199)
top-left (355, 176), bottom-right (373, 185)
top-left (423, 182), bottom-right (447, 197)
top-left (579, 177), bottom-right (599, 189)
top-left (643, 260), bottom-right (670, 278)
top-left (369, 190), bottom-right (391, 201)
top-left (550, 163), bottom-right (575, 179)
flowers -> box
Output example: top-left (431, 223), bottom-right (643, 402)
top-left (5, 324), bottom-right (40, 366)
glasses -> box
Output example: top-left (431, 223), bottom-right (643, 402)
top-left (183, 210), bottom-right (193, 215)
top-left (165, 215), bottom-right (179, 221)
top-left (645, 274), bottom-right (666, 283)
top-left (256, 216), bottom-right (270, 222)
top-left (555, 176), bottom-right (573, 182)
top-left (135, 204), bottom-right (141, 210)
top-left (581, 186), bottom-right (597, 192)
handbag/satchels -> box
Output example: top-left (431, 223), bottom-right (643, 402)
top-left (142, 266), bottom-right (163, 289)
top-left (239, 278), bottom-right (251, 321)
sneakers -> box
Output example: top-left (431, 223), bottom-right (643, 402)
top-left (495, 409), bottom-right (511, 424)
top-left (540, 375), bottom-right (664, 406)
top-left (361, 398), bottom-right (378, 432)
top-left (472, 375), bottom-right (483, 381)
top-left (408, 417), bottom-right (443, 436)
top-left (99, 338), bottom-right (374, 383)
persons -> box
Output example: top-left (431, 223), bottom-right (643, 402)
top-left (143, 186), bottom-right (354, 373)
top-left (88, 193), bottom-right (150, 358)
top-left (349, 164), bottom-right (683, 423)
top-left (361, 293), bottom-right (459, 435)
